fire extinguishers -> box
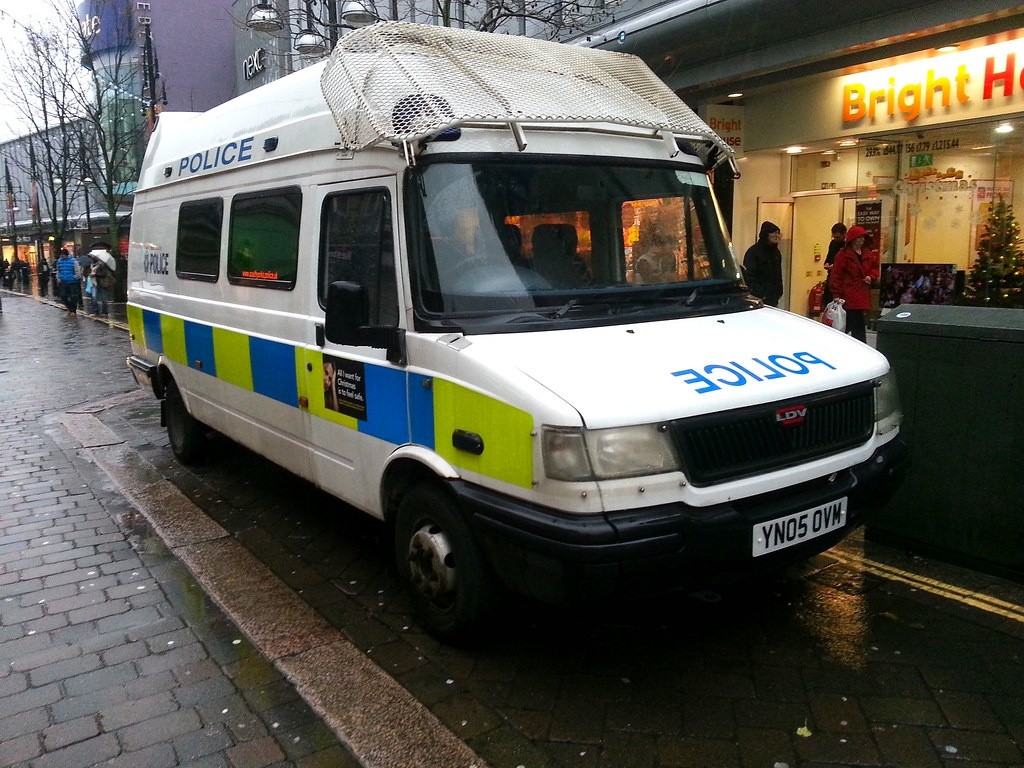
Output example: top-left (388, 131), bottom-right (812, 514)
top-left (808, 282), bottom-right (824, 318)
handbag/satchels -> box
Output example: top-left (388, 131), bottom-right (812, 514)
top-left (68, 311), bottom-right (76, 318)
top-left (100, 268), bottom-right (117, 288)
top-left (101, 313), bottom-right (106, 318)
top-left (821, 298), bottom-right (847, 332)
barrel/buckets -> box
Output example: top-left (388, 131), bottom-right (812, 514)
top-left (98, 301), bottom-right (113, 317)
top-left (107, 303), bottom-right (126, 321)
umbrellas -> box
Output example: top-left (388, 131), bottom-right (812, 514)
top-left (77, 255), bottom-right (97, 267)
top-left (88, 250), bottom-right (116, 271)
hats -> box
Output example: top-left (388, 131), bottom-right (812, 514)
top-left (845, 226), bottom-right (869, 242)
top-left (59, 249), bottom-right (69, 256)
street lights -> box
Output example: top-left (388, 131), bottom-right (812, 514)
top-left (246, 0), bottom-right (379, 55)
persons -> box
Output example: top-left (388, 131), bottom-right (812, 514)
top-left (824, 223), bottom-right (879, 344)
top-left (0, 249), bottom-right (109, 319)
top-left (634, 233), bottom-right (678, 282)
top-left (743, 220), bottom-right (784, 308)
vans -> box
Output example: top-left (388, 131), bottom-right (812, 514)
top-left (124, 20), bottom-right (909, 651)
